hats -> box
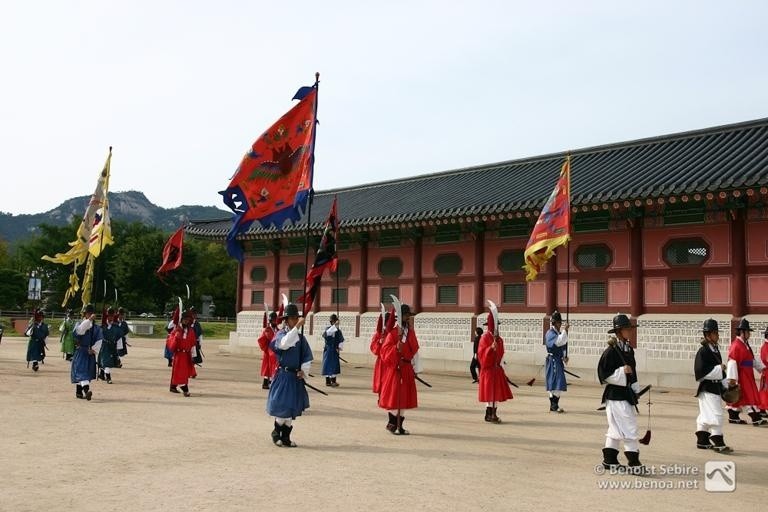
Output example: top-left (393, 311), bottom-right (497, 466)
top-left (381, 312), bottom-right (398, 325)
top-left (280, 304), bottom-right (304, 320)
top-left (31, 305), bottom-right (126, 316)
top-left (169, 305), bottom-right (200, 319)
top-left (482, 311), bottom-right (501, 325)
top-left (549, 311), bottom-right (563, 322)
top-left (699, 319), bottom-right (720, 334)
top-left (607, 314), bottom-right (639, 333)
top-left (395, 304), bottom-right (416, 317)
top-left (330, 314), bottom-right (339, 321)
top-left (268, 313), bottom-right (276, 320)
top-left (736, 319), bottom-right (753, 332)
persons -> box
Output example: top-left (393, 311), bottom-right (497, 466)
top-left (470, 327), bottom-right (483, 384)
top-left (165, 306), bottom-right (203, 396)
top-left (370, 313), bottom-right (393, 404)
top-left (24, 307), bottom-right (129, 400)
top-left (478, 314), bottom-right (513, 422)
top-left (380, 305), bottom-right (419, 436)
top-left (695, 320), bottom-right (768, 452)
top-left (266, 304), bottom-right (314, 447)
top-left (323, 314), bottom-right (344, 386)
top-left (545, 312), bottom-right (570, 413)
top-left (598, 316), bottom-right (646, 474)
top-left (258, 312), bottom-right (278, 389)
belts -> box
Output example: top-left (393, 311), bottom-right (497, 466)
top-left (627, 382), bottom-right (632, 387)
top-left (703, 379), bottom-right (722, 384)
top-left (175, 348), bottom-right (191, 353)
top-left (278, 364), bottom-right (300, 372)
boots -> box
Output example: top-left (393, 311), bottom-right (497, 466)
top-left (728, 408), bottom-right (747, 424)
top-left (83, 385), bottom-right (93, 401)
top-left (262, 377), bottom-right (272, 389)
top-left (397, 415), bottom-right (409, 434)
top-left (602, 447), bottom-right (630, 472)
top-left (75, 384), bottom-right (84, 399)
top-left (709, 434), bottom-right (734, 453)
top-left (281, 424), bottom-right (297, 447)
top-left (180, 385), bottom-right (190, 397)
top-left (624, 450), bottom-right (649, 475)
top-left (169, 384), bottom-right (180, 393)
top-left (748, 412), bottom-right (768, 427)
top-left (272, 419), bottom-right (282, 447)
top-left (325, 376), bottom-right (339, 387)
top-left (695, 430), bottom-right (713, 449)
top-left (385, 412), bottom-right (400, 434)
top-left (485, 406), bottom-right (501, 424)
top-left (550, 395), bottom-right (564, 413)
top-left (31, 354), bottom-right (123, 385)
top-left (760, 409), bottom-right (768, 418)
top-left (168, 358), bottom-right (197, 379)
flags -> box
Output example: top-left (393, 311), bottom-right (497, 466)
top-left (521, 160), bottom-right (572, 281)
top-left (157, 225), bottom-right (183, 285)
top-left (296, 198), bottom-right (339, 314)
top-left (218, 81), bottom-right (319, 262)
top-left (39, 152), bottom-right (111, 307)
